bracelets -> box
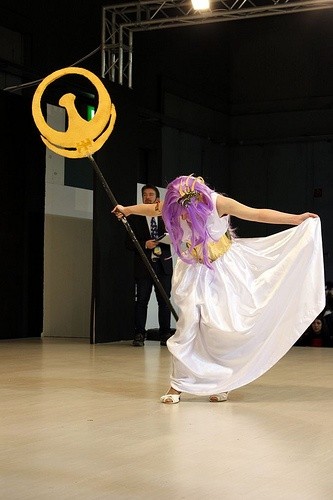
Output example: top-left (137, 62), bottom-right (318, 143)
top-left (145, 241), bottom-right (148, 250)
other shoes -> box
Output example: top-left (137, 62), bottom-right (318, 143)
top-left (134, 333), bottom-right (145, 346)
top-left (160, 334), bottom-right (169, 346)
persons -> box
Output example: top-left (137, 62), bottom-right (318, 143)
top-left (126, 185), bottom-right (173, 347)
top-left (111, 173), bottom-right (326, 405)
top-left (302, 317), bottom-right (333, 347)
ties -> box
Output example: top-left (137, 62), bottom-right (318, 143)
top-left (149, 216), bottom-right (160, 241)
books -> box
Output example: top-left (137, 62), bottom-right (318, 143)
top-left (151, 232), bottom-right (173, 245)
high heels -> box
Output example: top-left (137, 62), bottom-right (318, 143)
top-left (159, 386), bottom-right (182, 404)
top-left (208, 389), bottom-right (232, 401)
